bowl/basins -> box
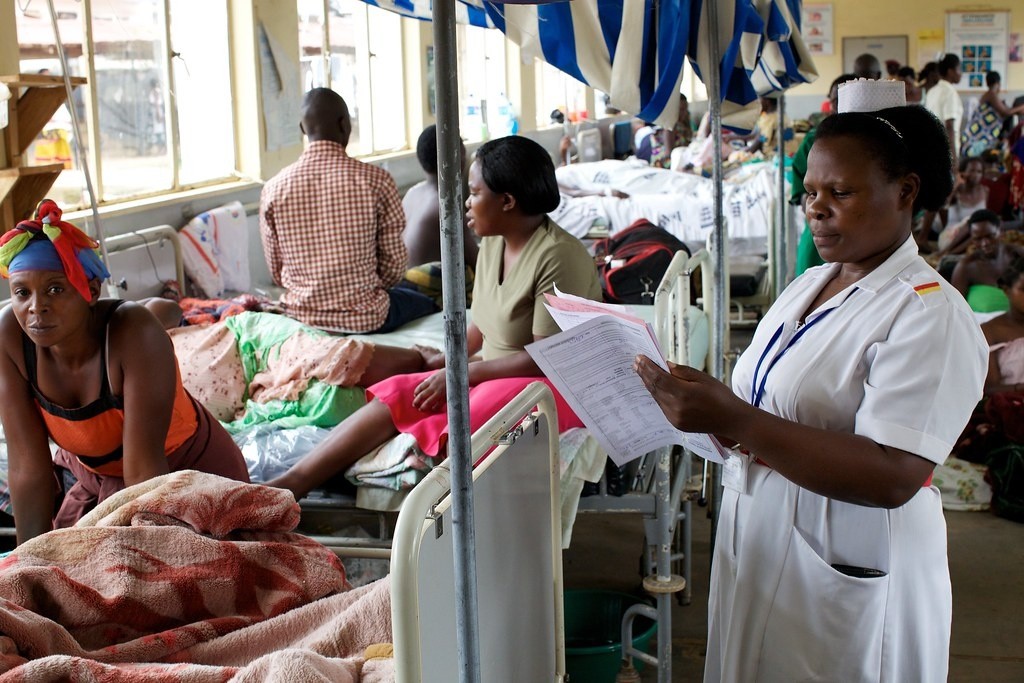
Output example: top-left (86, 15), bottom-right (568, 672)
top-left (563, 588), bottom-right (659, 683)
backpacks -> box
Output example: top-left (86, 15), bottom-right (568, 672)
top-left (592, 217), bottom-right (697, 308)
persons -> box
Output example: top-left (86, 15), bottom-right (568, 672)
top-left (787, 53), bottom-right (1024, 523)
top-left (259, 88), bottom-right (480, 336)
top-left (550, 93), bottom-right (798, 169)
top-left (258, 135), bottom-right (602, 502)
top-left (136, 298), bottom-right (482, 427)
top-left (0, 200), bottom-right (251, 548)
top-left (634, 77), bottom-right (989, 683)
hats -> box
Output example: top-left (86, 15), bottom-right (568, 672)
top-left (837, 77), bottom-right (907, 113)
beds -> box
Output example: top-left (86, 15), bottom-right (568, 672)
top-left (0, 110), bottom-right (805, 683)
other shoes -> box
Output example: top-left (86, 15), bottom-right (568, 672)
top-left (915, 227), bottom-right (939, 243)
top-left (916, 244), bottom-right (933, 256)
top-left (939, 209), bottom-right (949, 228)
